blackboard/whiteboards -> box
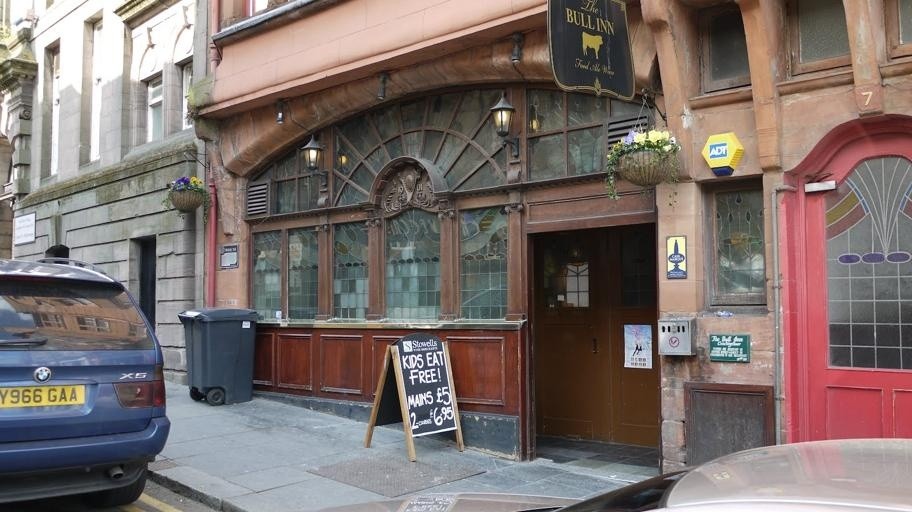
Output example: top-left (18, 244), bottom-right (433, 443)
top-left (393, 332), bottom-right (458, 437)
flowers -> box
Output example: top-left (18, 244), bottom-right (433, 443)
top-left (159, 176), bottom-right (212, 225)
top-left (602, 128), bottom-right (683, 212)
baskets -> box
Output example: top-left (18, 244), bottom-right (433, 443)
top-left (170, 189), bottom-right (204, 212)
top-left (617, 149), bottom-right (670, 186)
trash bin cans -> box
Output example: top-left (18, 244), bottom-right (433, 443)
top-left (177, 307), bottom-right (258, 407)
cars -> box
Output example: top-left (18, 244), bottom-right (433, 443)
top-left (0, 256), bottom-right (171, 510)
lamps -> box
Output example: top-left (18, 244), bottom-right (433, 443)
top-left (301, 134), bottom-right (329, 188)
top-left (488, 89), bottom-right (519, 158)
top-left (276, 100), bottom-right (285, 124)
top-left (511, 32), bottom-right (523, 64)
top-left (377, 72), bottom-right (387, 100)
